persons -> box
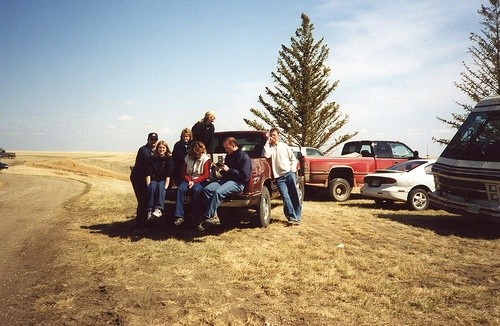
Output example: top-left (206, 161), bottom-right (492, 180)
top-left (130, 133), bottom-right (158, 225)
top-left (146, 141), bottom-right (174, 221)
top-left (408, 151), bottom-right (423, 160)
top-left (191, 111), bottom-right (215, 162)
top-left (197, 137), bottom-right (252, 231)
top-left (174, 141), bottom-right (211, 225)
top-left (172, 128), bottom-right (193, 187)
top-left (261, 128), bottom-right (302, 226)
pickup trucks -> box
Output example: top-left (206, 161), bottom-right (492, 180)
top-left (300, 139), bottom-right (423, 202)
top-left (165, 129), bottom-right (305, 228)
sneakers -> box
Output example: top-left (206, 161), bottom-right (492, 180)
top-left (197, 217), bottom-right (221, 232)
top-left (147, 209), bottom-right (163, 220)
top-left (173, 217), bottom-right (185, 231)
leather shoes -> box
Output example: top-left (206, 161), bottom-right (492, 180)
top-left (287, 216), bottom-right (301, 225)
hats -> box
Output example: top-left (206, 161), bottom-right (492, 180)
top-left (147, 132), bottom-right (161, 139)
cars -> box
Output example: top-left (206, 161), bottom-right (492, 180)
top-left (288, 145), bottom-right (327, 158)
top-left (360, 158), bottom-right (438, 211)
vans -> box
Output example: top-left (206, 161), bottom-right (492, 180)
top-left (423, 96), bottom-right (500, 223)
